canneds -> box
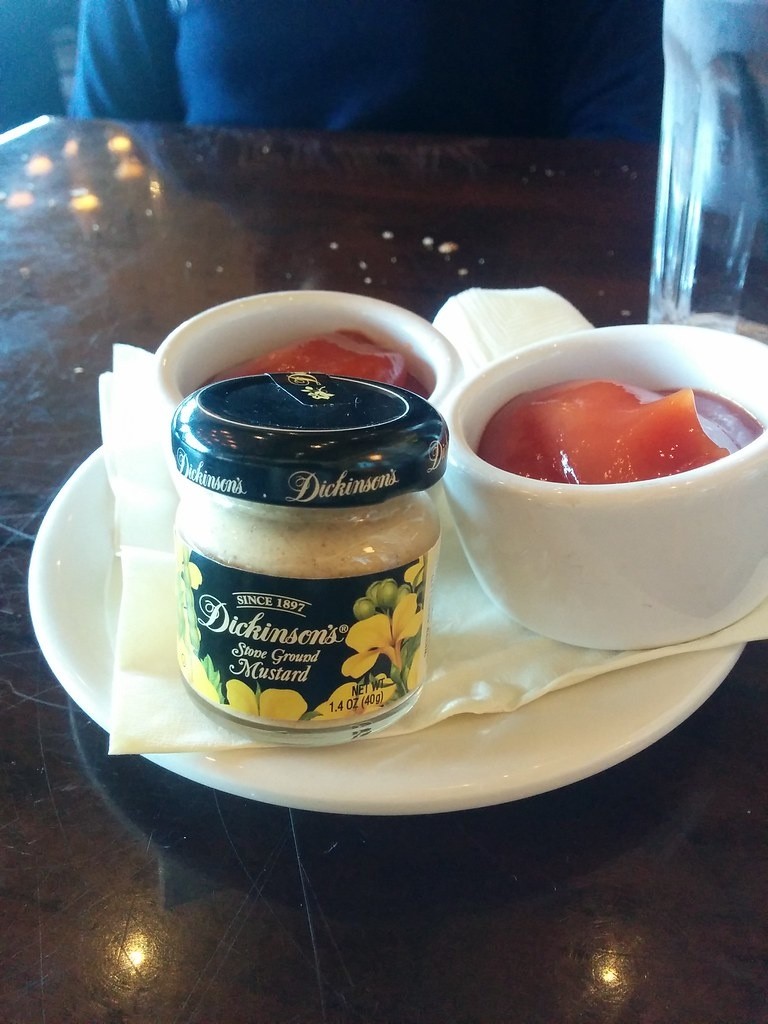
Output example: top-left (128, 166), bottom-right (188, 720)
top-left (167, 374), bottom-right (450, 746)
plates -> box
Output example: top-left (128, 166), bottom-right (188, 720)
top-left (26, 452), bottom-right (749, 814)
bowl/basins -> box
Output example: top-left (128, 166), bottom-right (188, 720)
top-left (153, 290), bottom-right (465, 454)
top-left (435, 323), bottom-right (768, 651)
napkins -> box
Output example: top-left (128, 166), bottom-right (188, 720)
top-left (97, 287), bottom-right (768, 758)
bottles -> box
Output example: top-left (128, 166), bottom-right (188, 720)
top-left (647, 0), bottom-right (768, 344)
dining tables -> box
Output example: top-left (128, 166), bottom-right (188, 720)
top-left (0, 113), bottom-right (767, 1024)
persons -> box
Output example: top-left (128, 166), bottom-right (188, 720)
top-left (67, 0), bottom-right (664, 147)
